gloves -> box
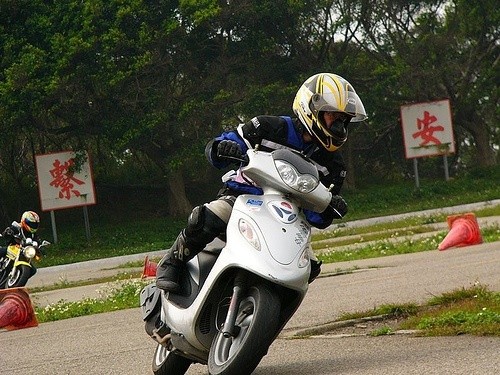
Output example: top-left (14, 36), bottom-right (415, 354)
top-left (330, 194), bottom-right (348, 219)
top-left (217, 140), bottom-right (242, 162)
top-left (2, 227), bottom-right (14, 237)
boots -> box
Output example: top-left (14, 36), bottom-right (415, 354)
top-left (155, 227), bottom-right (201, 291)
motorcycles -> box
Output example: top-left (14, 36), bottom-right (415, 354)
top-left (0, 231), bottom-right (51, 288)
top-left (141, 141), bottom-right (348, 374)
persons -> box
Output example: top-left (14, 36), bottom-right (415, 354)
top-left (0, 209), bottom-right (40, 262)
top-left (156, 73), bottom-right (367, 296)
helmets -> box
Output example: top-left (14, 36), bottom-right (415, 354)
top-left (292, 73), bottom-right (369, 152)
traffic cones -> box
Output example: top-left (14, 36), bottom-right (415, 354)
top-left (1, 288), bottom-right (39, 329)
top-left (145, 256), bottom-right (160, 277)
top-left (438, 213), bottom-right (480, 250)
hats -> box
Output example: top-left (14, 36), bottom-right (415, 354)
top-left (21, 211), bottom-right (40, 233)
